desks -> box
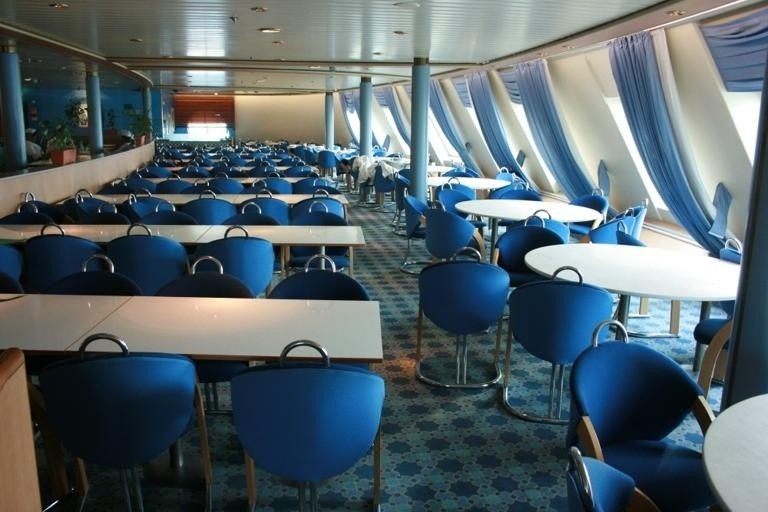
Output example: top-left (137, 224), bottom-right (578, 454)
top-left (525, 244), bottom-right (744, 371)
top-left (702, 393), bottom-right (767, 512)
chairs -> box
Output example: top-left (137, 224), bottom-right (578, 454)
top-left (565, 323), bottom-right (713, 510)
top-left (229, 338), bottom-right (385, 511)
top-left (418, 247), bottom-right (513, 391)
top-left (502, 264), bottom-right (630, 423)
top-left (272, 140), bottom-right (651, 276)
top-left (0, 140), bottom-right (373, 301)
top-left (40, 334), bottom-right (214, 510)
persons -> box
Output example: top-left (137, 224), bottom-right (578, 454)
top-left (113, 128), bottom-right (136, 151)
top-left (23, 127), bottom-right (45, 163)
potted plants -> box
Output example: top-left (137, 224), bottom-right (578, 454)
top-left (46, 103), bottom-right (79, 166)
top-left (102, 109), bottom-right (120, 140)
top-left (128, 107), bottom-right (153, 144)
